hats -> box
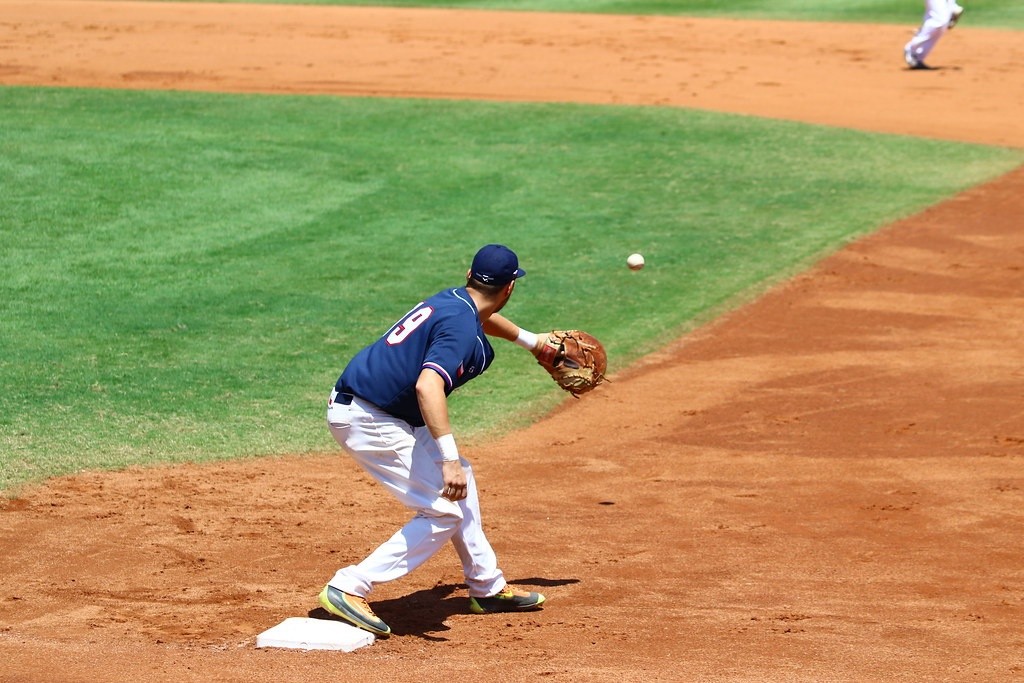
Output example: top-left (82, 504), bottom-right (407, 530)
top-left (471, 244), bottom-right (526, 286)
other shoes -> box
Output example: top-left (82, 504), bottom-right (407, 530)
top-left (904, 45), bottom-right (930, 70)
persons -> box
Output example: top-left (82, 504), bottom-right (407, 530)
top-left (905, 0), bottom-right (955, 68)
top-left (318, 244), bottom-right (608, 635)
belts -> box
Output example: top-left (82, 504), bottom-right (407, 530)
top-left (333, 393), bottom-right (353, 406)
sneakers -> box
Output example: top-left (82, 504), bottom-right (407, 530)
top-left (316, 584), bottom-right (392, 636)
top-left (469, 582), bottom-right (546, 613)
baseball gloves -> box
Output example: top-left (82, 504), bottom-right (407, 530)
top-left (534, 331), bottom-right (607, 394)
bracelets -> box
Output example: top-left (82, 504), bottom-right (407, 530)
top-left (515, 328), bottom-right (537, 350)
top-left (435, 433), bottom-right (459, 461)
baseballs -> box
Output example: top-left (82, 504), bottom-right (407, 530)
top-left (627, 253), bottom-right (645, 270)
